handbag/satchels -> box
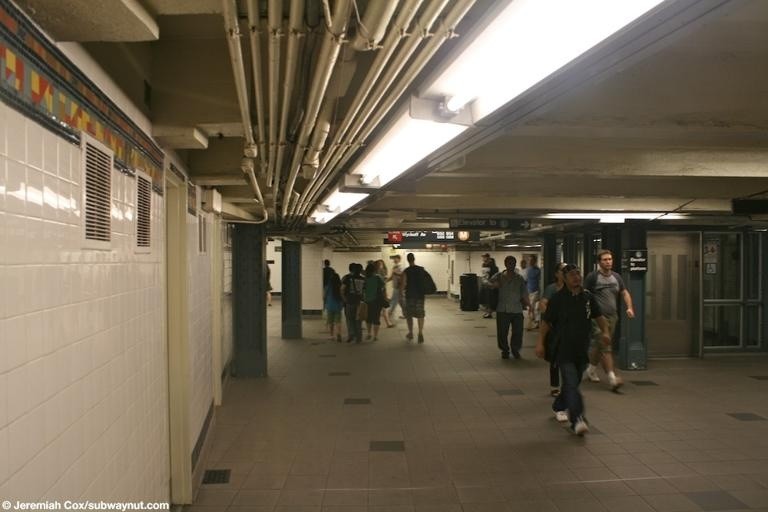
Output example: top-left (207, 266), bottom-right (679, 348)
top-left (423, 271), bottom-right (437, 295)
top-left (377, 288), bottom-right (390, 308)
top-left (347, 293), bottom-right (360, 305)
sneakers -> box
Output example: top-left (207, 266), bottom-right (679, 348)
top-left (608, 378), bottom-right (624, 390)
top-left (552, 389), bottom-right (560, 397)
top-left (551, 408), bottom-right (569, 423)
top-left (574, 419), bottom-right (589, 435)
top-left (587, 368), bottom-right (600, 382)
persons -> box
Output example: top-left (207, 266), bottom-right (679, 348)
top-left (480, 249), bottom-right (635, 435)
top-left (322, 253), bottom-right (437, 344)
top-left (266, 263), bottom-right (272, 306)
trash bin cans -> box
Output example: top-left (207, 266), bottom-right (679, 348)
top-left (459, 273), bottom-right (479, 311)
